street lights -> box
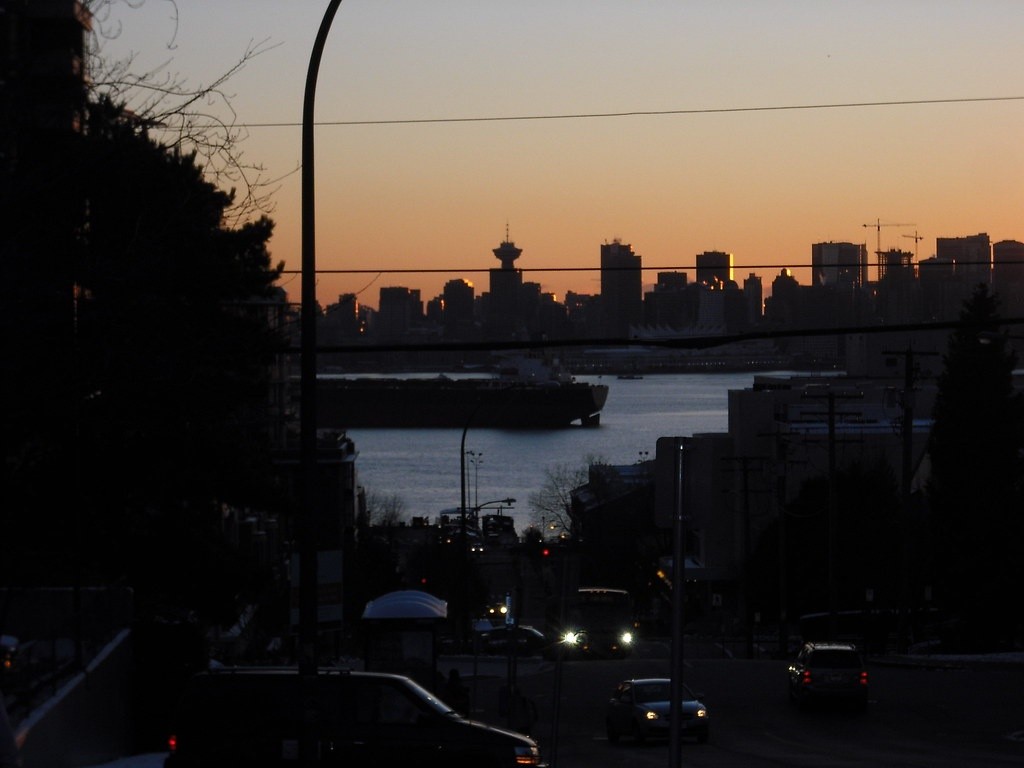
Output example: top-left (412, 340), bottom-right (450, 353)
top-left (461, 379), bottom-right (561, 562)
top-left (465, 498), bottom-right (517, 522)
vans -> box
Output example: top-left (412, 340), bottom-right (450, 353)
top-left (154, 665), bottom-right (538, 766)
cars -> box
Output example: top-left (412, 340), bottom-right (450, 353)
top-left (604, 675), bottom-right (721, 747)
top-left (790, 639), bottom-right (882, 722)
top-left (550, 583), bottom-right (650, 662)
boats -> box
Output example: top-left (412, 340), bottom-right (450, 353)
top-left (297, 358), bottom-right (610, 431)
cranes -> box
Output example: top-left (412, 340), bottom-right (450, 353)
top-left (863, 218), bottom-right (924, 282)
